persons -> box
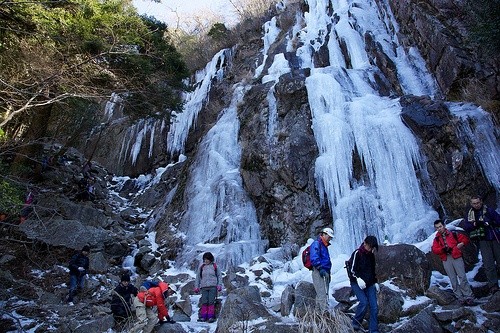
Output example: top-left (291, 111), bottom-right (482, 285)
top-left (107, 274), bottom-right (138, 333)
top-left (431, 220), bottom-right (475, 306)
top-left (460, 195), bottom-right (500, 295)
top-left (128, 281), bottom-right (177, 333)
top-left (348, 235), bottom-right (380, 333)
top-left (194, 252), bottom-right (222, 323)
top-left (310, 228), bottom-right (333, 319)
top-left (67, 245), bottom-right (90, 308)
top-left (0, 154), bottom-right (97, 224)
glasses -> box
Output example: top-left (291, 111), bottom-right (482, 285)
top-left (169, 290), bottom-right (174, 294)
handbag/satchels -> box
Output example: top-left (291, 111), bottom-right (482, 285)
top-left (470, 225), bottom-right (488, 239)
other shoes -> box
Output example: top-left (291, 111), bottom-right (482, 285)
top-left (351, 324), bottom-right (360, 331)
top-left (490, 286), bottom-right (499, 295)
top-left (458, 300), bottom-right (472, 306)
top-left (209, 317), bottom-right (214, 323)
top-left (198, 318), bottom-right (205, 322)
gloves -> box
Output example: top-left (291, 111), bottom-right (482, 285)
top-left (357, 277), bottom-right (366, 289)
top-left (160, 321), bottom-right (166, 325)
top-left (217, 285), bottom-right (223, 292)
top-left (442, 247), bottom-right (453, 254)
top-left (194, 287), bottom-right (200, 293)
top-left (375, 283), bottom-right (380, 294)
top-left (325, 272), bottom-right (331, 283)
top-left (165, 316), bottom-right (171, 321)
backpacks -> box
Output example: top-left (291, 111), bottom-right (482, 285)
top-left (140, 279), bottom-right (158, 291)
top-left (302, 240), bottom-right (322, 271)
top-left (344, 249), bottom-right (363, 282)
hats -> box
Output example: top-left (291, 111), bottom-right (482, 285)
top-left (322, 227), bottom-right (334, 238)
top-left (121, 275), bottom-right (130, 281)
top-left (365, 235), bottom-right (378, 251)
top-left (168, 284), bottom-right (178, 295)
